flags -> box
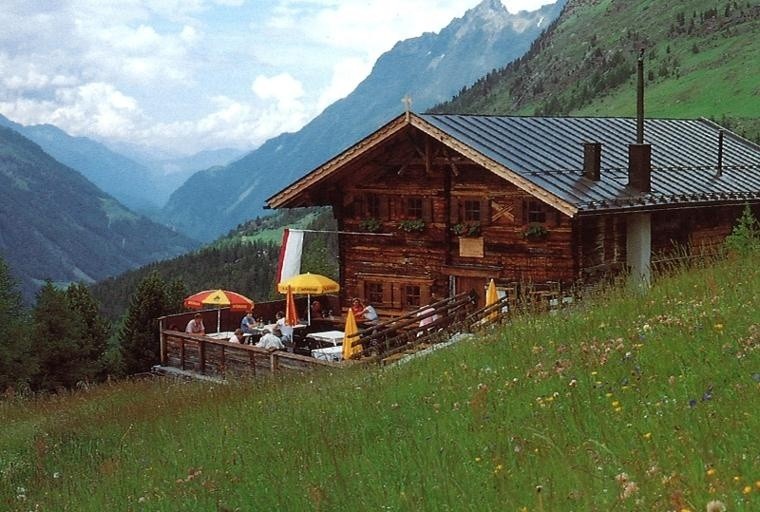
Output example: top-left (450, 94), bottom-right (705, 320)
top-left (275, 229), bottom-right (305, 283)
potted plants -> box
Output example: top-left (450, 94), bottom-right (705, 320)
top-left (360, 219), bottom-right (551, 243)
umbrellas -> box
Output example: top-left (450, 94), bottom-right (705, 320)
top-left (484, 278), bottom-right (503, 329)
top-left (277, 270), bottom-right (341, 326)
top-left (342, 306), bottom-right (364, 360)
top-left (285, 283), bottom-right (301, 328)
top-left (183, 289), bottom-right (255, 334)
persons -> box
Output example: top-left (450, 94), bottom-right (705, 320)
top-left (256, 329), bottom-right (286, 350)
top-left (273, 311), bottom-right (292, 343)
top-left (416, 300), bottom-right (438, 339)
top-left (352, 297), bottom-right (363, 318)
top-left (240, 312), bottom-right (257, 333)
top-left (354, 297), bottom-right (378, 321)
top-left (183, 312), bottom-right (206, 338)
top-left (303, 300), bottom-right (322, 321)
top-left (228, 328), bottom-right (244, 345)
